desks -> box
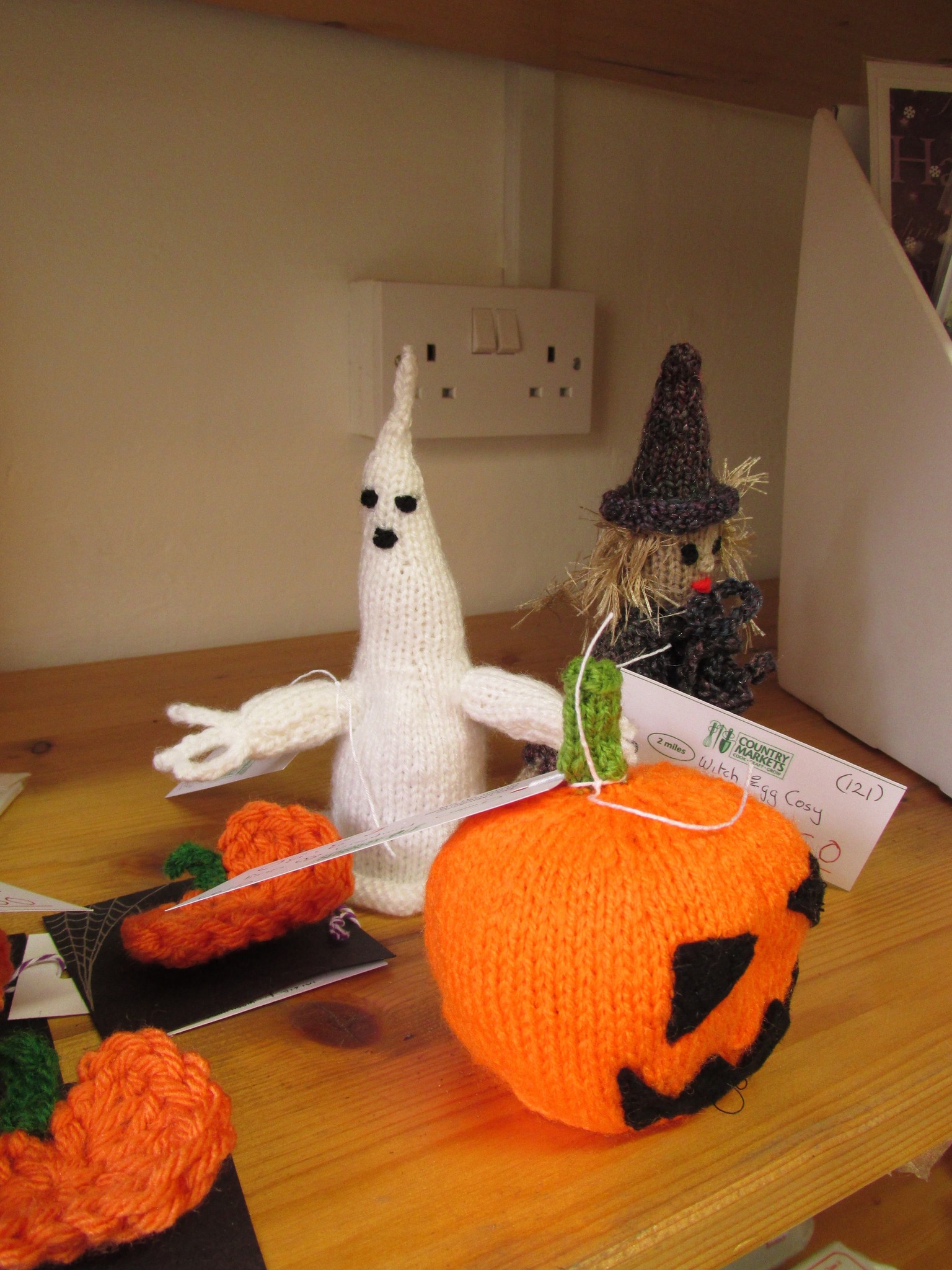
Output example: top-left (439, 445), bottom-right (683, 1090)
top-left (0, 576), bottom-right (951, 1270)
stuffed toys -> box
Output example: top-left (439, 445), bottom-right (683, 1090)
top-left (581, 340), bottom-right (771, 744)
top-left (149, 336), bottom-right (572, 921)
top-left (426, 652), bottom-right (829, 1145)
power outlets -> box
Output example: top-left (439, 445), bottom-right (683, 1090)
top-left (347, 280), bottom-right (596, 439)
